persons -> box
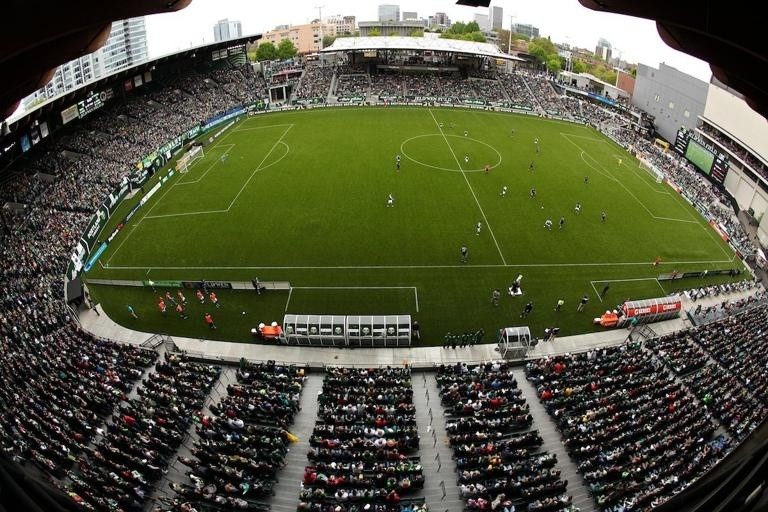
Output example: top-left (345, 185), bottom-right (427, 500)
top-left (0, 57), bottom-right (768, 512)
